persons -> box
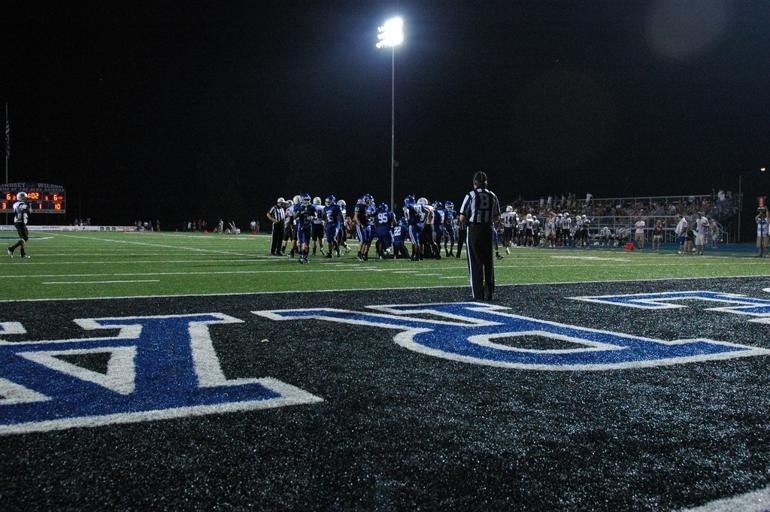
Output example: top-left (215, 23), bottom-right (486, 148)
top-left (491, 189), bottom-right (735, 255)
top-left (136, 219), bottom-right (256, 234)
top-left (67, 215), bottom-right (93, 226)
top-left (5, 191), bottom-right (31, 259)
top-left (458, 172), bottom-right (503, 303)
top-left (755, 212), bottom-right (770, 258)
top-left (266, 193), bottom-right (467, 262)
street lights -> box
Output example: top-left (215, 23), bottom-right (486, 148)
top-left (376, 16), bottom-right (404, 214)
top-left (738, 167), bottom-right (766, 241)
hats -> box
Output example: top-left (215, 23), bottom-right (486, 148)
top-left (474, 172), bottom-right (487, 182)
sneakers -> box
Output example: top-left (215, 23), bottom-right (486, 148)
top-left (376, 251), bottom-right (455, 260)
top-left (497, 249), bottom-right (510, 259)
top-left (271, 246), bottom-right (352, 263)
top-left (356, 252), bottom-right (369, 261)
top-left (465, 298), bottom-right (496, 302)
top-left (7, 248), bottom-right (31, 259)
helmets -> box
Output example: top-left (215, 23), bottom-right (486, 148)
top-left (505, 205), bottom-right (587, 221)
top-left (277, 194), bottom-right (346, 208)
top-left (362, 195), bottom-right (394, 222)
top-left (403, 195), bottom-right (454, 209)
top-left (398, 217), bottom-right (408, 227)
top-left (16, 191), bottom-right (26, 201)
